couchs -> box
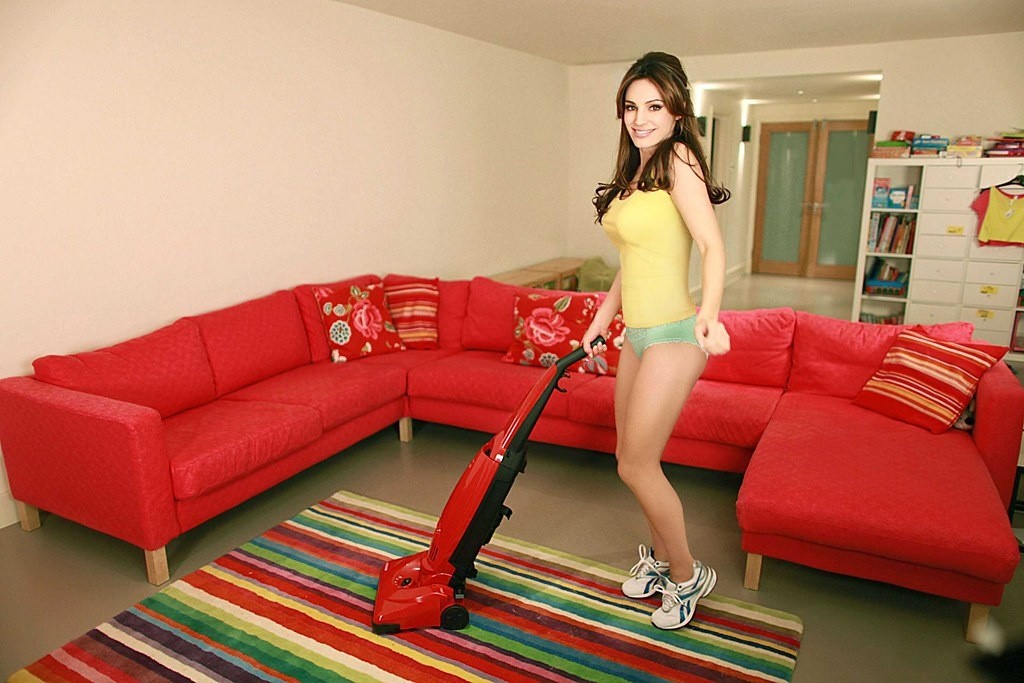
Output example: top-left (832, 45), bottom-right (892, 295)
top-left (0, 271), bottom-right (1024, 645)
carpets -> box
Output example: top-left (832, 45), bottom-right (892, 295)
top-left (7, 489), bottom-right (804, 683)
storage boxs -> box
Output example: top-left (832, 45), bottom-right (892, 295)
top-left (866, 133), bottom-right (983, 158)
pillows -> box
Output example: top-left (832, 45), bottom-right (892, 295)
top-left (312, 278), bottom-right (441, 364)
top-left (852, 325), bottom-right (1011, 436)
top-left (500, 290), bottom-right (625, 376)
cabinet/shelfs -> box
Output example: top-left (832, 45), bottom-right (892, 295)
top-left (489, 257), bottom-right (586, 291)
top-left (851, 157), bottom-right (1024, 347)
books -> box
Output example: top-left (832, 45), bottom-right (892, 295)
top-left (860, 177), bottom-right (920, 326)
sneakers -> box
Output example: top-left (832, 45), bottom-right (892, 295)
top-left (651, 560), bottom-right (718, 630)
top-left (622, 544), bottom-right (670, 597)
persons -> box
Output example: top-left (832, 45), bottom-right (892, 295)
top-left (581, 52), bottom-right (731, 630)
